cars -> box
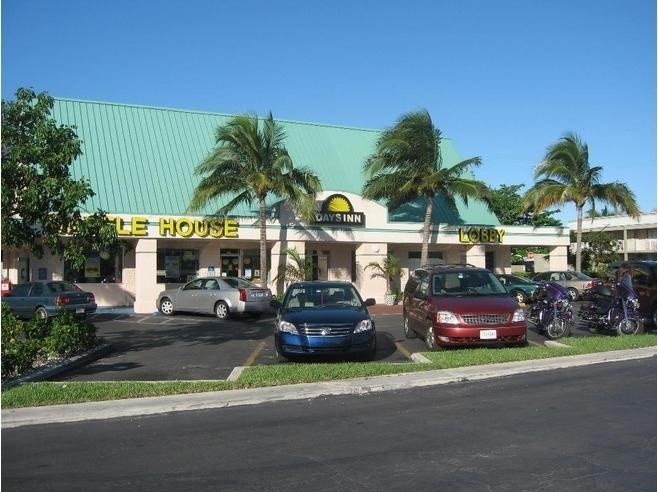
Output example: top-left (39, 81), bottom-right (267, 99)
top-left (270, 281), bottom-right (375, 361)
top-left (403, 264), bottom-right (527, 351)
top-left (156, 277), bottom-right (272, 320)
top-left (1, 280), bottom-right (97, 323)
top-left (607, 260), bottom-right (657, 332)
top-left (494, 271), bottom-right (602, 302)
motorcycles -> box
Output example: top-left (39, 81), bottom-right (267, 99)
top-left (577, 281), bottom-right (640, 336)
top-left (527, 282), bottom-right (576, 340)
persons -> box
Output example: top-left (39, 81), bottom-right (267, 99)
top-left (619, 262), bottom-right (633, 289)
